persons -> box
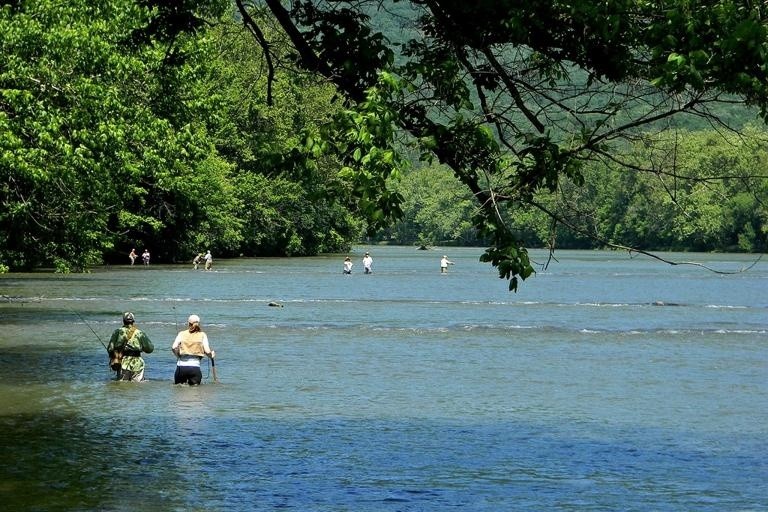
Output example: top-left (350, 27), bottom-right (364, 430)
top-left (440, 254), bottom-right (456, 273)
top-left (141, 248), bottom-right (151, 265)
top-left (128, 248), bottom-right (139, 266)
top-left (200, 249), bottom-right (214, 271)
top-left (105, 311), bottom-right (155, 381)
top-left (362, 251), bottom-right (374, 274)
top-left (191, 253), bottom-right (204, 270)
top-left (342, 256), bottom-right (354, 274)
top-left (170, 313), bottom-right (216, 386)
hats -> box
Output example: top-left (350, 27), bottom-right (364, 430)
top-left (365, 252), bottom-right (368, 255)
top-left (187, 314), bottom-right (202, 324)
top-left (123, 312), bottom-right (135, 322)
top-left (345, 256), bottom-right (349, 260)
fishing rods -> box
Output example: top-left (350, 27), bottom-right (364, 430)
top-left (67, 306), bottom-right (108, 351)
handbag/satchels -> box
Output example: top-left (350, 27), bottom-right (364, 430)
top-left (109, 349), bottom-right (124, 372)
